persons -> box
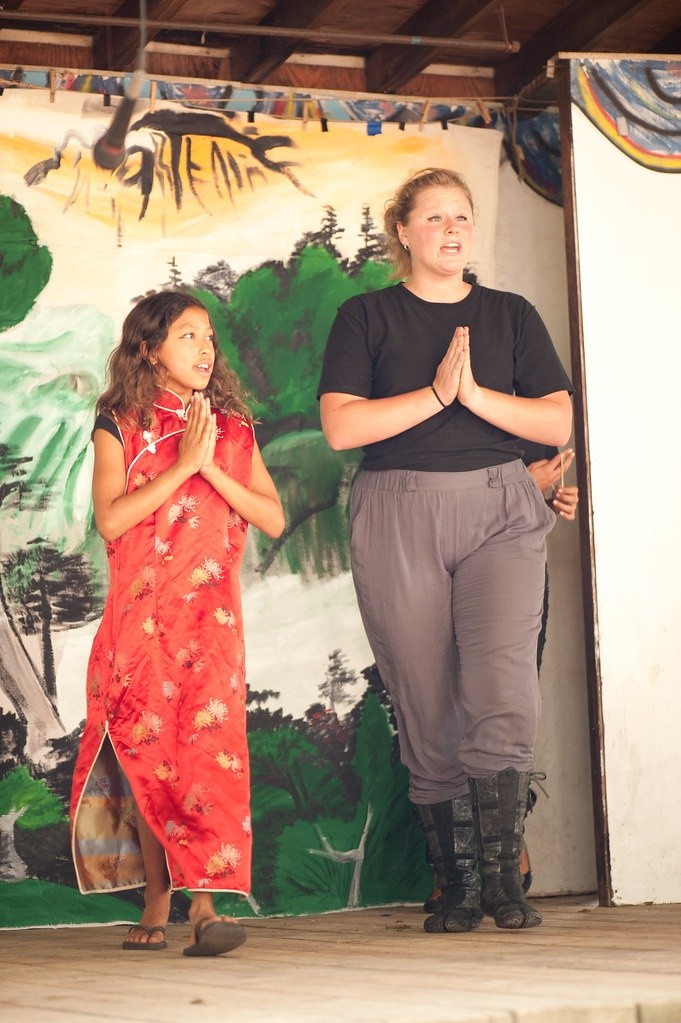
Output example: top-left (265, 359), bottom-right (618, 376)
top-left (413, 437), bottom-right (579, 912)
top-left (69, 289), bottom-right (287, 956)
top-left (311, 162), bottom-right (574, 934)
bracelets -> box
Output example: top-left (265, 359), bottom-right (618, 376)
top-left (429, 384), bottom-right (452, 413)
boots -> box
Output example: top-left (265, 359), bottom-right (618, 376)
top-left (466, 768), bottom-right (544, 929)
top-left (412, 792), bottom-right (486, 933)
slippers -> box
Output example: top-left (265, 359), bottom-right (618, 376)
top-left (182, 916), bottom-right (248, 956)
top-left (122, 923), bottom-right (167, 950)
